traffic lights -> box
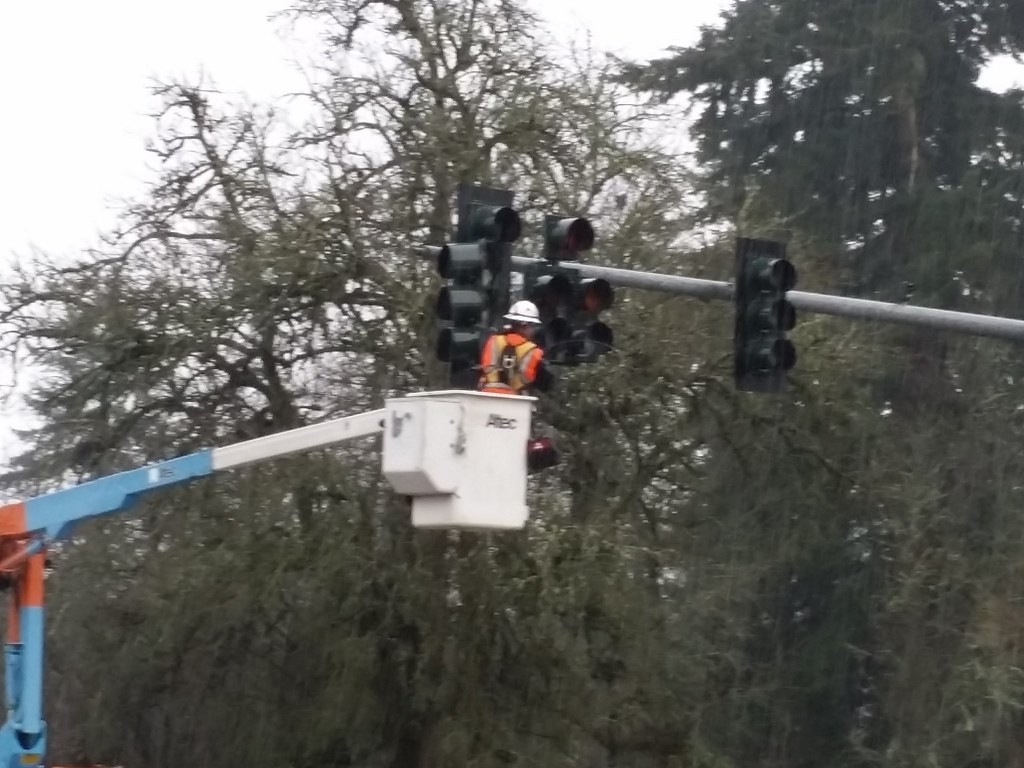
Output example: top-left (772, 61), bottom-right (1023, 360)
top-left (524, 262), bottom-right (574, 367)
top-left (733, 237), bottom-right (798, 392)
top-left (541, 214), bottom-right (596, 261)
top-left (563, 267), bottom-right (614, 363)
top-left (471, 200), bottom-right (525, 244)
top-left (431, 240), bottom-right (491, 387)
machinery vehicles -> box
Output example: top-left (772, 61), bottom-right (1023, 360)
top-left (1, 384), bottom-right (541, 768)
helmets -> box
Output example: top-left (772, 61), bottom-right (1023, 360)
top-left (502, 300), bottom-right (543, 325)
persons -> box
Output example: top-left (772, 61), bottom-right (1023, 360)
top-left (465, 300), bottom-right (561, 475)
